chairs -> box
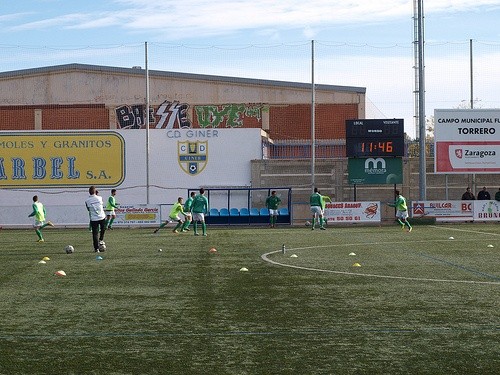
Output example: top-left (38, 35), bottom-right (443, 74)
top-left (219, 208), bottom-right (229, 216)
top-left (239, 208), bottom-right (249, 216)
top-left (249, 208), bottom-right (260, 216)
top-left (204, 208), bottom-right (209, 216)
top-left (260, 208), bottom-right (269, 216)
top-left (279, 207), bottom-right (289, 216)
top-left (229, 208), bottom-right (239, 217)
top-left (210, 208), bottom-right (220, 216)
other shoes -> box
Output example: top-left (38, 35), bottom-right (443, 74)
top-left (48, 221), bottom-right (54, 227)
top-left (181, 230), bottom-right (187, 232)
top-left (95, 249), bottom-right (100, 253)
top-left (312, 227), bottom-right (314, 230)
top-left (88, 230), bottom-right (92, 232)
top-left (401, 224), bottom-right (404, 230)
top-left (320, 227), bottom-right (325, 230)
top-left (173, 230), bottom-right (179, 233)
top-left (38, 239), bottom-right (44, 242)
top-left (108, 226), bottom-right (113, 230)
top-left (203, 234), bottom-right (207, 236)
top-left (194, 234), bottom-right (199, 236)
top-left (325, 219), bottom-right (327, 225)
top-left (184, 228), bottom-right (190, 231)
top-left (409, 227), bottom-right (412, 232)
top-left (271, 224), bottom-right (275, 228)
top-left (154, 230), bottom-right (158, 233)
top-left (99, 240), bottom-right (105, 244)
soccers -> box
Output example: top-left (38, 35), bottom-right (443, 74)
top-left (66, 245), bottom-right (74, 254)
top-left (305, 221), bottom-right (310, 226)
top-left (98, 241), bottom-right (106, 251)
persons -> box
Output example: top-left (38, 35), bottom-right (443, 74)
top-left (461, 186), bottom-right (475, 222)
top-left (386, 189), bottom-right (413, 232)
top-left (189, 186), bottom-right (208, 236)
top-left (105, 188), bottom-right (118, 229)
top-left (309, 186), bottom-right (332, 230)
top-left (88, 190), bottom-right (102, 232)
top-left (266, 190), bottom-right (281, 228)
top-left (28, 194), bottom-right (55, 242)
top-left (477, 186), bottom-right (491, 221)
top-left (153, 196), bottom-right (191, 233)
top-left (85, 185), bottom-right (105, 252)
top-left (180, 191), bottom-right (196, 232)
top-left (494, 187), bottom-right (499, 223)
